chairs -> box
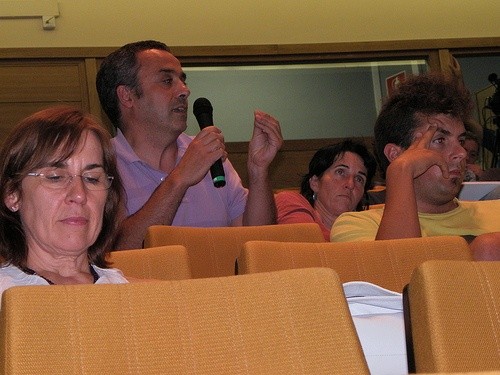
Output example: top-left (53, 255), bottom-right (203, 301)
top-left (0, 186), bottom-right (500, 375)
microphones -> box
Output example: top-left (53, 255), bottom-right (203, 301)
top-left (192, 98), bottom-right (226, 188)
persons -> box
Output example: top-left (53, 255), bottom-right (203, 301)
top-left (275, 141), bottom-right (377, 242)
top-left (330, 76), bottom-right (500, 261)
top-left (450, 57), bottom-right (500, 169)
top-left (462, 136), bottom-right (486, 181)
top-left (0, 105), bottom-right (127, 295)
top-left (96, 40), bottom-right (282, 250)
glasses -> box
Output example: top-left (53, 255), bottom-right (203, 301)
top-left (14, 167), bottom-right (114, 191)
